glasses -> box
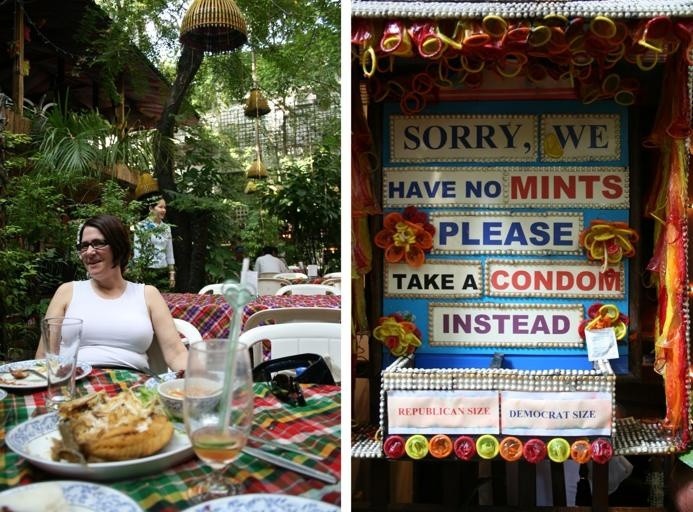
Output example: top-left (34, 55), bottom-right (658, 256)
top-left (74, 240), bottom-right (108, 253)
top-left (269, 374), bottom-right (306, 408)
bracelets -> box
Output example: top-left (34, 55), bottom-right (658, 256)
top-left (168, 268), bottom-right (176, 275)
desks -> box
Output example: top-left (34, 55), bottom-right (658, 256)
top-left (352, 420), bottom-right (692, 511)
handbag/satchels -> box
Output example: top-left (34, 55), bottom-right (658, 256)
top-left (249, 353), bottom-right (336, 389)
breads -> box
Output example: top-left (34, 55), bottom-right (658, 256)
top-left (58, 385), bottom-right (175, 460)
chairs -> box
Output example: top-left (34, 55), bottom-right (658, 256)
top-left (235, 324), bottom-right (341, 382)
top-left (147, 319), bottom-right (206, 376)
top-left (241, 308), bottom-right (341, 366)
top-left (196, 266), bottom-right (341, 295)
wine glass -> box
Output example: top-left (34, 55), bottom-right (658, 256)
top-left (183, 339), bottom-right (254, 505)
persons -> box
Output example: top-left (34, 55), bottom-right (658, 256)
top-left (33, 214), bottom-right (204, 376)
top-left (271, 246), bottom-right (288, 270)
top-left (254, 246), bottom-right (285, 273)
top-left (132, 193), bottom-right (176, 290)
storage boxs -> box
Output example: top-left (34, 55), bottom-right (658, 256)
top-left (379, 354), bottom-right (617, 436)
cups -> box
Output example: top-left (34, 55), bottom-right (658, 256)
top-left (40, 316), bottom-right (83, 413)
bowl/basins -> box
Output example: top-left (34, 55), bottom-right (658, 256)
top-left (157, 378), bottom-right (223, 418)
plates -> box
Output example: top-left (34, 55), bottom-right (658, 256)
top-left (180, 493), bottom-right (341, 511)
top-left (144, 371), bottom-right (242, 394)
top-left (0, 358), bottom-right (94, 388)
top-left (0, 481), bottom-right (144, 512)
top-left (4, 412), bottom-right (194, 480)
